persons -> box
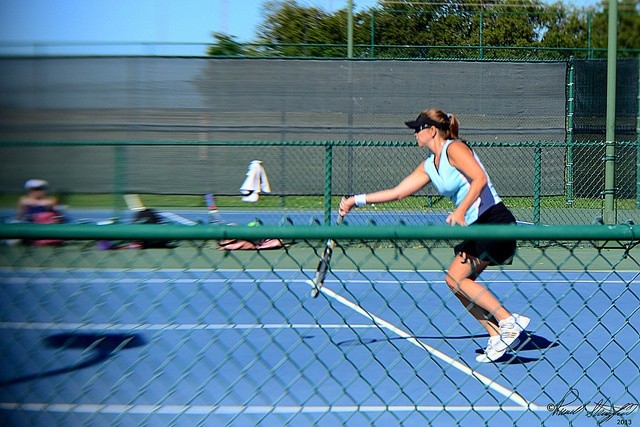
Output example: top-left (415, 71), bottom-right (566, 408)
top-left (18, 179), bottom-right (57, 244)
top-left (339, 108), bottom-right (531, 362)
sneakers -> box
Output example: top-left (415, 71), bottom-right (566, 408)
top-left (476, 336), bottom-right (521, 363)
top-left (497, 313), bottom-right (531, 352)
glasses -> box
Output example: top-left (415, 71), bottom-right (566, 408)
top-left (415, 124), bottom-right (431, 133)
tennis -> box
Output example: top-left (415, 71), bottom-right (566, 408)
top-left (248, 222), bottom-right (259, 228)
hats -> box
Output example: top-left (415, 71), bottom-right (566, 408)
top-left (404, 115), bottom-right (449, 130)
top-left (24, 180), bottom-right (50, 190)
